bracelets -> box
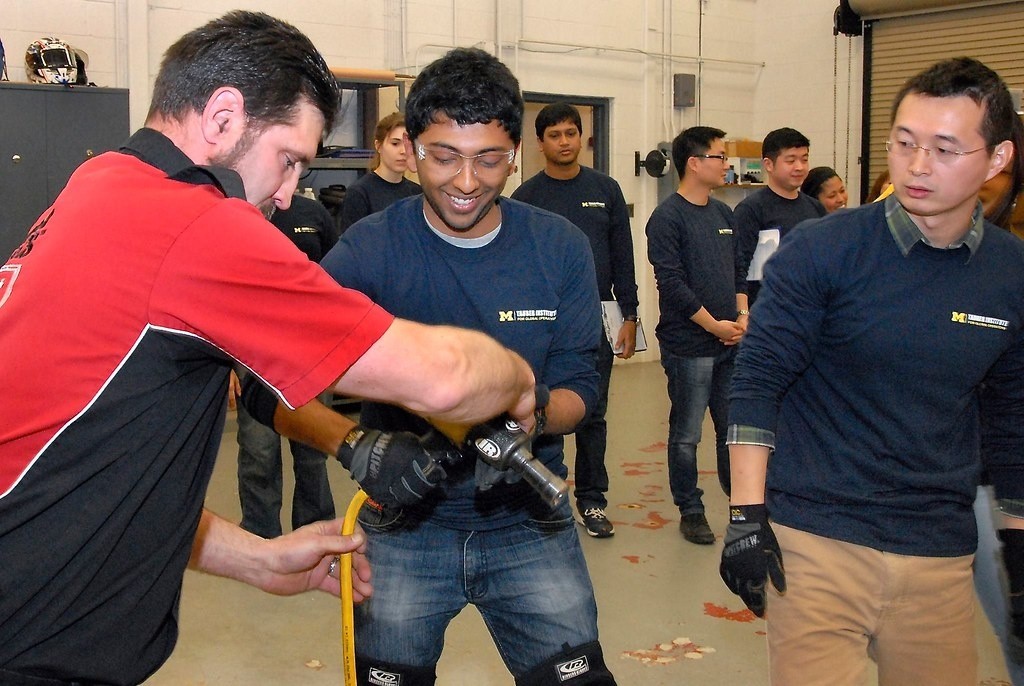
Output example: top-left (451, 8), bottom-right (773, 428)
top-left (738, 310), bottom-right (749, 315)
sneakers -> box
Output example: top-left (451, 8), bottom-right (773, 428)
top-left (679, 512), bottom-right (716, 543)
top-left (577, 507), bottom-right (615, 536)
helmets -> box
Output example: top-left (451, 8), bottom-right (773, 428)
top-left (25, 36), bottom-right (89, 85)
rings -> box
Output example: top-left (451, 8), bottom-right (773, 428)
top-left (329, 559), bottom-right (339, 576)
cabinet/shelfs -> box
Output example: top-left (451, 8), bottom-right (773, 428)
top-left (0, 80), bottom-right (131, 269)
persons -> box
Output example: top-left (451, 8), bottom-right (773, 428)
top-left (511, 102), bottom-right (639, 537)
top-left (801, 167), bottom-right (848, 214)
top-left (228, 194), bottom-right (340, 539)
top-left (733, 127), bottom-right (828, 309)
top-left (321, 47), bottom-right (617, 686)
top-left (720, 56), bottom-right (1024, 686)
top-left (645, 126), bottom-right (748, 544)
top-left (0, 11), bottom-right (536, 686)
top-left (341, 112), bottom-right (424, 236)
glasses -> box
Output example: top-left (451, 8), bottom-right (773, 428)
top-left (413, 140), bottom-right (515, 178)
top-left (694, 155), bottom-right (729, 164)
top-left (885, 139), bottom-right (997, 164)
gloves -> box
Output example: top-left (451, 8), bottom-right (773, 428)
top-left (472, 403), bottom-right (545, 490)
top-left (335, 425), bottom-right (447, 509)
top-left (719, 504), bottom-right (787, 618)
top-left (997, 528), bottom-right (1024, 636)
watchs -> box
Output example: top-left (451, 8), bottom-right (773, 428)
top-left (623, 316), bottom-right (641, 326)
